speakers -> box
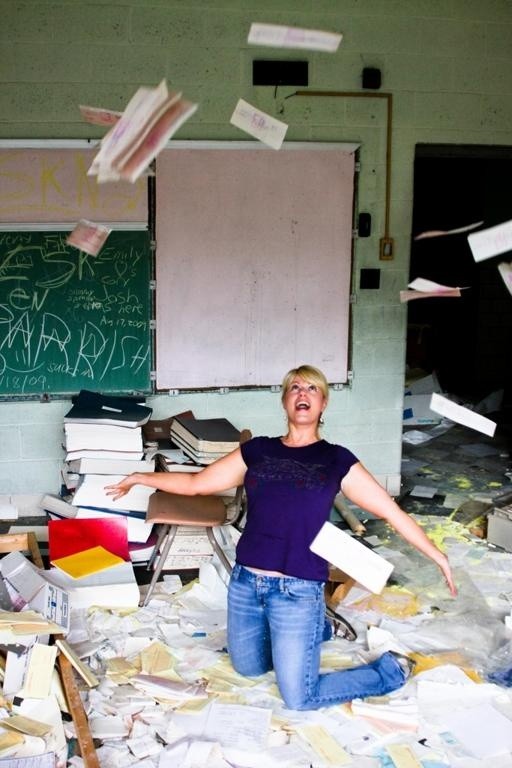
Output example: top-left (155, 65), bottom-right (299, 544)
top-left (362, 67), bottom-right (381, 88)
top-left (252, 59), bottom-right (309, 87)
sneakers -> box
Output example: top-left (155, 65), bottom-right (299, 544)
top-left (325, 606), bottom-right (358, 642)
top-left (388, 650), bottom-right (415, 681)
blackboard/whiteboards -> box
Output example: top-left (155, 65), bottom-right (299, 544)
top-left (1, 145), bottom-right (150, 229)
top-left (0, 227), bottom-right (152, 398)
top-left (150, 141), bottom-right (356, 393)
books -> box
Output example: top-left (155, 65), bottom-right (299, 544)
top-left (86, 79), bottom-right (199, 182)
top-left (1, 389), bottom-right (512, 767)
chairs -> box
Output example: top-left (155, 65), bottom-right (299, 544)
top-left (140, 429), bottom-right (253, 607)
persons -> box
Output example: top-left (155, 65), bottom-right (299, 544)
top-left (103, 364), bottom-right (457, 713)
